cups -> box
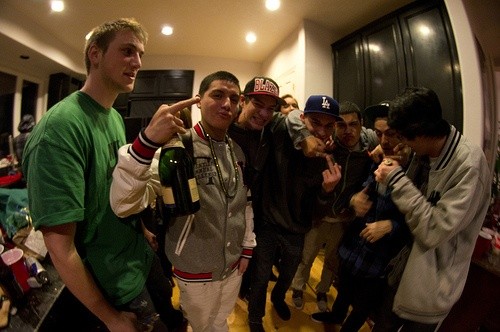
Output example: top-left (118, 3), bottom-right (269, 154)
top-left (481, 228), bottom-right (495, 237)
top-left (474, 232), bottom-right (492, 260)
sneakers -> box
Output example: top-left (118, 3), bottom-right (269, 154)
top-left (317, 290), bottom-right (328, 312)
top-left (310, 313), bottom-right (341, 325)
top-left (291, 287), bottom-right (305, 310)
top-left (273, 298), bottom-right (292, 321)
top-left (249, 318), bottom-right (266, 332)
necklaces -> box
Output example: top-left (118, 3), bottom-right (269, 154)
top-left (206, 133), bottom-right (240, 198)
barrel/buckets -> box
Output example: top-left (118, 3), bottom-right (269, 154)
top-left (1, 248), bottom-right (30, 292)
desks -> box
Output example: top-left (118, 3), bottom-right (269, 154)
top-left (0, 167), bottom-right (67, 332)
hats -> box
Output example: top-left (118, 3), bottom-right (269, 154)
top-left (18, 114), bottom-right (36, 133)
top-left (360, 99), bottom-right (394, 121)
top-left (243, 77), bottom-right (285, 106)
top-left (304, 95), bottom-right (345, 122)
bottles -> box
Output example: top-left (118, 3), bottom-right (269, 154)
top-left (158, 141), bottom-right (178, 217)
top-left (167, 133), bottom-right (200, 216)
top-left (26, 255), bottom-right (49, 284)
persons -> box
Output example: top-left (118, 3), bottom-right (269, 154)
top-left (278, 93), bottom-right (300, 117)
top-left (12, 115), bottom-right (36, 169)
top-left (281, 101), bottom-right (428, 332)
top-left (20, 18), bottom-right (187, 331)
top-left (373, 84), bottom-right (494, 332)
top-left (241, 95), bottom-right (345, 332)
top-left (107, 70), bottom-right (259, 332)
top-left (222, 75), bottom-right (290, 304)
